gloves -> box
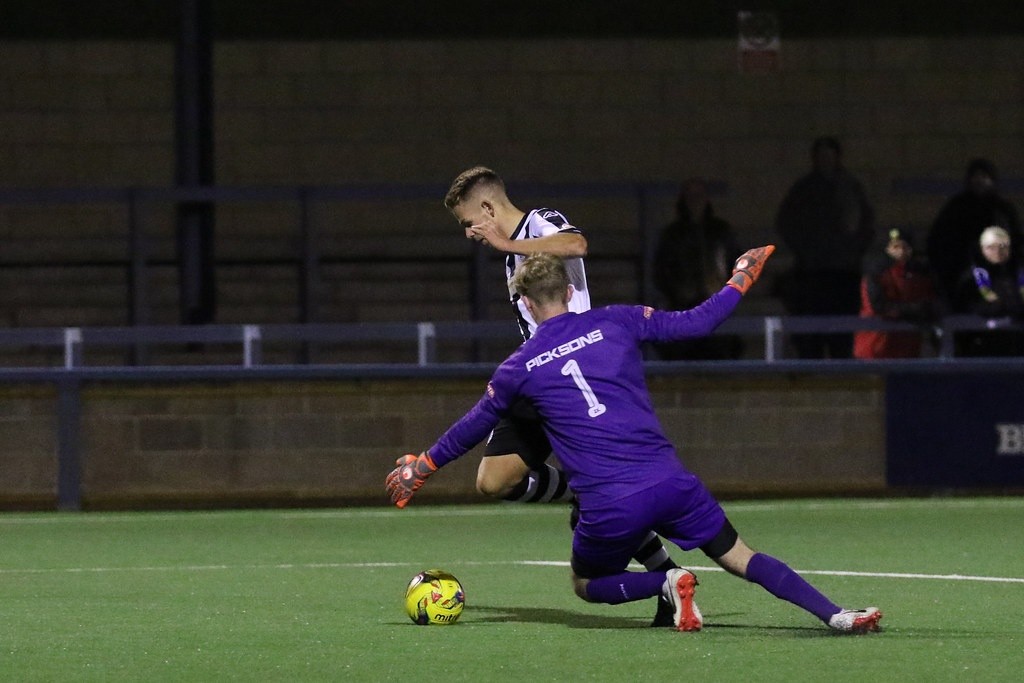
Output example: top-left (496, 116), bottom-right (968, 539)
top-left (384, 450), bottom-right (441, 510)
top-left (726, 245), bottom-right (776, 297)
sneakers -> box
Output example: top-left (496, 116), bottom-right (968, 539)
top-left (652, 568), bottom-right (699, 627)
top-left (662, 570), bottom-right (704, 631)
top-left (829, 607), bottom-right (882, 633)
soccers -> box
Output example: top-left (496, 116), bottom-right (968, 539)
top-left (405, 568), bottom-right (465, 626)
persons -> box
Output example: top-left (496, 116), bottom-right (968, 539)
top-left (444, 166), bottom-right (699, 628)
top-left (383, 242), bottom-right (881, 636)
top-left (947, 226), bottom-right (1023, 358)
top-left (652, 177), bottom-right (750, 360)
top-left (772, 135), bottom-right (877, 360)
top-left (924, 156), bottom-right (1024, 310)
top-left (852, 225), bottom-right (931, 360)
